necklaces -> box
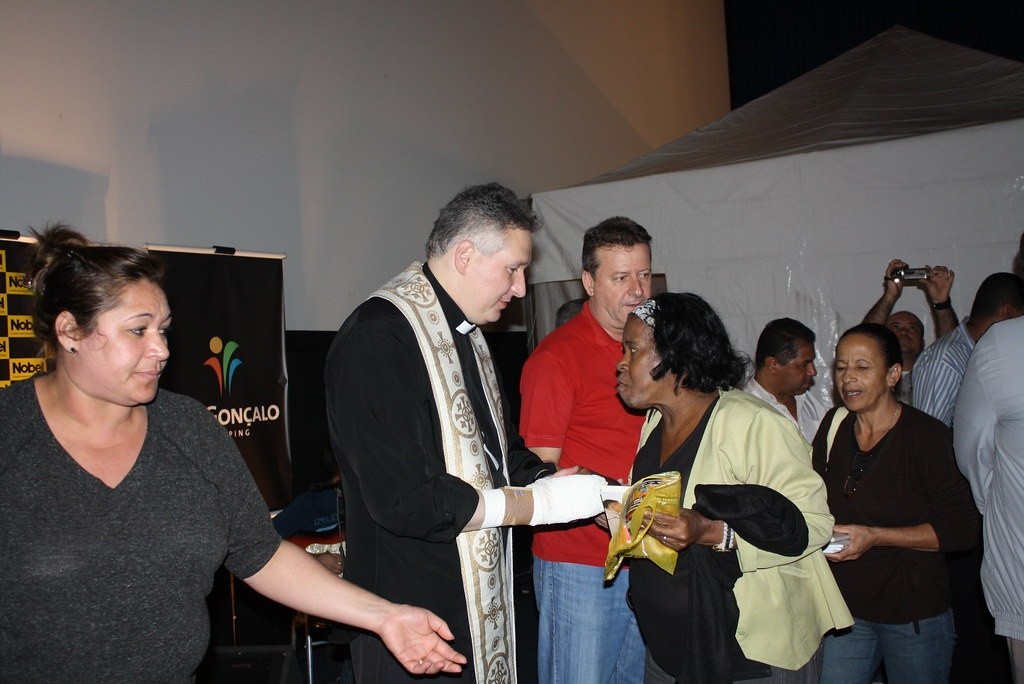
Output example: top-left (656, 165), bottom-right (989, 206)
top-left (854, 404), bottom-right (900, 451)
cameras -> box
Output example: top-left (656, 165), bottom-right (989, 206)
top-left (890, 264), bottom-right (928, 283)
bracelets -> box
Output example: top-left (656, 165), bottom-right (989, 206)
top-left (713, 522), bottom-right (735, 552)
top-left (930, 297), bottom-right (952, 311)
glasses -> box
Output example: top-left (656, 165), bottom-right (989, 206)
top-left (843, 449), bottom-right (874, 501)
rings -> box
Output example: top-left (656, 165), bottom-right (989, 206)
top-left (663, 536), bottom-right (667, 545)
top-left (420, 658), bottom-right (426, 663)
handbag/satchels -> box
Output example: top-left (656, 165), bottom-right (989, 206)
top-left (604, 471), bottom-right (682, 585)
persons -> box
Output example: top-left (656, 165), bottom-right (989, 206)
top-left (324, 183), bottom-right (610, 684)
top-left (862, 233), bottom-right (1024, 684)
top-left (738, 315), bottom-right (827, 445)
top-left (812, 322), bottom-right (985, 684)
top-left (613, 292), bottom-right (858, 684)
top-left (517, 216), bottom-right (652, 684)
top-left (0, 223), bottom-right (468, 684)
